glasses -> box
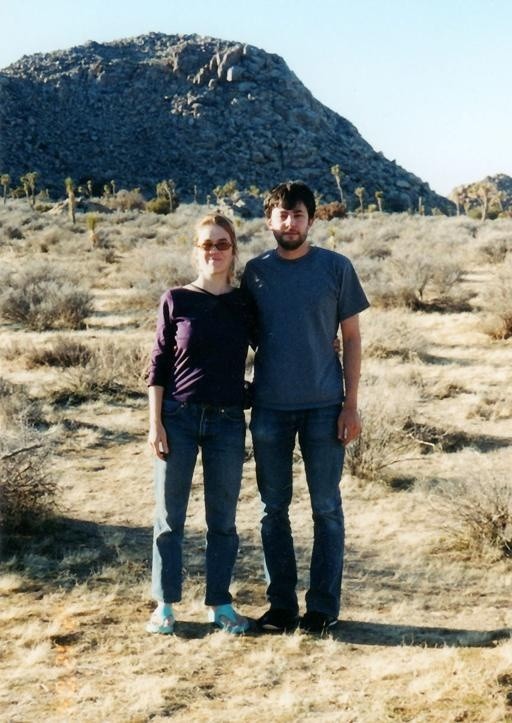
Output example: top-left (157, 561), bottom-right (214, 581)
top-left (197, 241), bottom-right (233, 250)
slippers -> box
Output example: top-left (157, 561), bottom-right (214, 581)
top-left (208, 604), bottom-right (248, 633)
top-left (147, 607), bottom-right (173, 633)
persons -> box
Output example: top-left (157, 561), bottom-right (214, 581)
top-left (144, 214), bottom-right (342, 636)
top-left (238, 180), bottom-right (371, 631)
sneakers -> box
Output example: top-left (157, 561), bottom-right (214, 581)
top-left (260, 610), bottom-right (337, 633)
